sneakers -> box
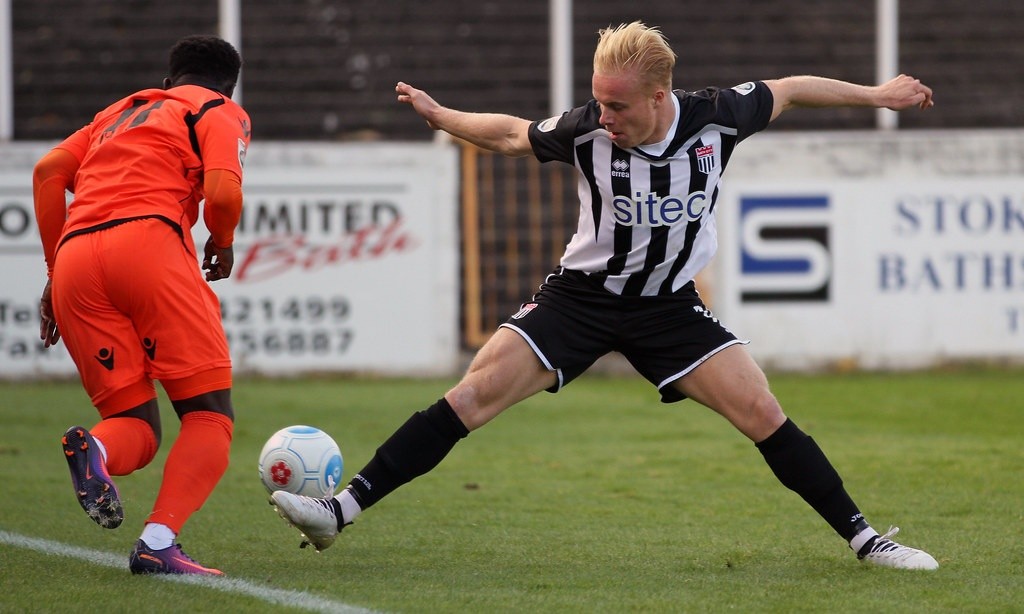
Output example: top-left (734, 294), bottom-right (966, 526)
top-left (269, 474), bottom-right (340, 553)
top-left (62, 426), bottom-right (123, 530)
top-left (128, 538), bottom-right (225, 577)
top-left (859, 525), bottom-right (939, 571)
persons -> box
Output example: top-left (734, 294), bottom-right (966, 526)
top-left (33, 35), bottom-right (252, 574)
top-left (271, 21), bottom-right (939, 571)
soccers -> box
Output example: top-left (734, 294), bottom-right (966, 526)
top-left (256, 423), bottom-right (346, 501)
